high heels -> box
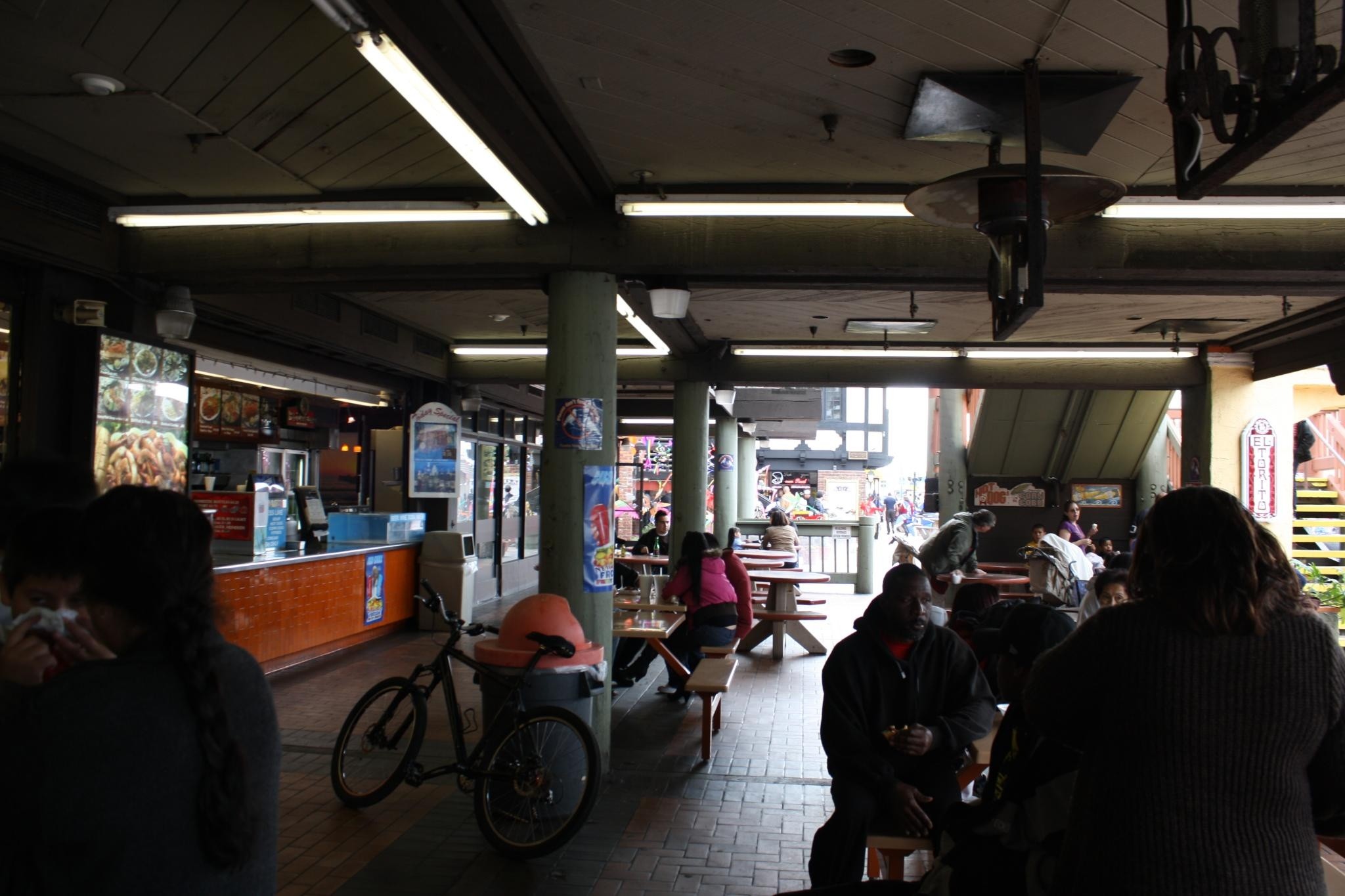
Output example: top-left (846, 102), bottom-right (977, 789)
top-left (619, 658), bottom-right (651, 682)
top-left (667, 688), bottom-right (692, 702)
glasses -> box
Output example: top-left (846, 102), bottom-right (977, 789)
top-left (1067, 508), bottom-right (1081, 513)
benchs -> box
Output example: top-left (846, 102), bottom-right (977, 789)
top-left (998, 591), bottom-right (1043, 599)
top-left (702, 632), bottom-right (744, 656)
top-left (863, 833), bottom-right (931, 880)
top-left (685, 654), bottom-right (740, 693)
top-left (748, 581), bottom-right (829, 623)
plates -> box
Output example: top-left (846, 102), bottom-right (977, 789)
top-left (618, 590), bottom-right (641, 594)
top-left (199, 393), bottom-right (260, 427)
top-left (104, 350), bottom-right (187, 421)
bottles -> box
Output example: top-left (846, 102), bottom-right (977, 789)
top-left (621, 544), bottom-right (625, 556)
top-left (653, 536), bottom-right (660, 556)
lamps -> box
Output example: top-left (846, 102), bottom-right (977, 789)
top-left (730, 344), bottom-right (963, 358)
top-left (147, 285), bottom-right (196, 340)
top-left (612, 183), bottom-right (1345, 228)
top-left (460, 384), bottom-right (483, 412)
top-left (450, 341), bottom-right (657, 358)
top-left (616, 294), bottom-right (672, 352)
top-left (740, 417), bottom-right (758, 433)
top-left (962, 345), bottom-right (1200, 361)
top-left (624, 275), bottom-right (693, 321)
top-left (107, 199), bottom-right (517, 228)
top-left (711, 383), bottom-right (737, 405)
top-left (347, 26), bottom-right (550, 230)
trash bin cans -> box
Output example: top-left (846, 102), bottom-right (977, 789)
top-left (417, 531), bottom-right (478, 631)
top-left (473, 594), bottom-right (604, 814)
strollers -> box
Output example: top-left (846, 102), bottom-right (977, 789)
top-left (1016, 534), bottom-right (1098, 609)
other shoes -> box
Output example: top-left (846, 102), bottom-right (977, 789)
top-left (658, 685), bottom-right (678, 694)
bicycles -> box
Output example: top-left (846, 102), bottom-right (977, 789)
top-left (330, 579), bottom-right (603, 860)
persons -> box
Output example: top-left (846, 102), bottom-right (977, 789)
top-left (614, 421), bottom-right (1344, 896)
top-left (1, 485), bottom-right (280, 896)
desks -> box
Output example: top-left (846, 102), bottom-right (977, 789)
top-left (978, 558), bottom-right (1028, 572)
top-left (741, 571), bottom-right (834, 660)
top-left (740, 541), bottom-right (802, 551)
top-left (613, 552), bottom-right (669, 575)
top-left (611, 582), bottom-right (688, 614)
top-left (937, 571), bottom-right (1031, 590)
top-left (611, 605), bottom-right (721, 760)
top-left (732, 548), bottom-right (796, 560)
top-left (741, 560), bottom-right (785, 570)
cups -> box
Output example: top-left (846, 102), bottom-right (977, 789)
top-left (204, 476), bottom-right (215, 491)
top-left (638, 574), bottom-right (653, 598)
top-left (590, 503), bottom-right (610, 545)
top-left (653, 575), bottom-right (670, 598)
top-left (393, 467), bottom-right (401, 480)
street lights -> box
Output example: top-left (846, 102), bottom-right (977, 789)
top-left (909, 472), bottom-right (923, 505)
